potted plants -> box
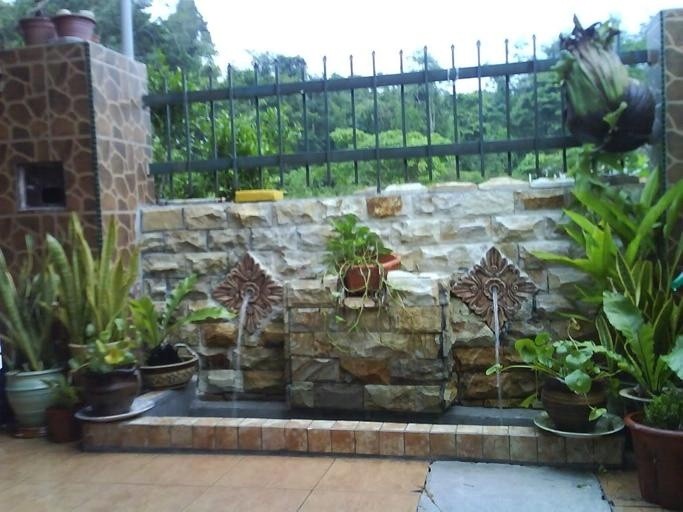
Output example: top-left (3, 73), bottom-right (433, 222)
top-left (318, 213), bottom-right (408, 352)
top-left (589, 290), bottom-right (683, 510)
top-left (3, 212), bottom-right (237, 441)
top-left (533, 144), bottom-right (683, 402)
top-left (488, 318), bottom-right (625, 438)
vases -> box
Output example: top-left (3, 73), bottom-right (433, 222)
top-left (19, 18), bottom-right (53, 45)
top-left (52, 15), bottom-right (95, 41)
